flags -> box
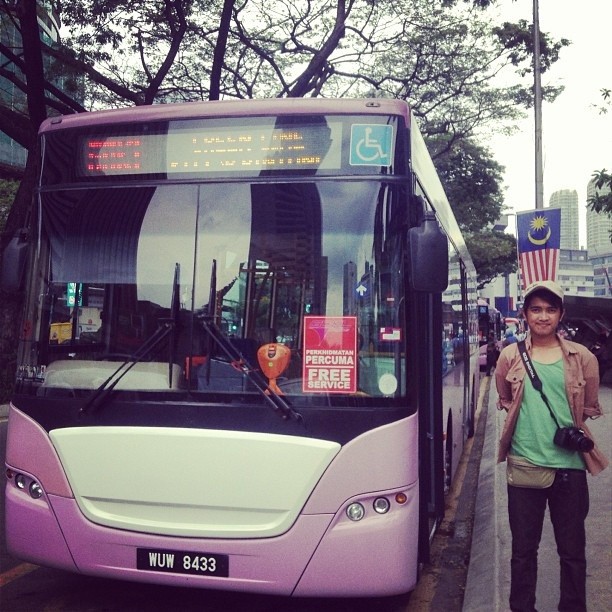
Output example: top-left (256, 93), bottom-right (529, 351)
top-left (516, 206), bottom-right (561, 289)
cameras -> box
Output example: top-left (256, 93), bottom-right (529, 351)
top-left (553, 425), bottom-right (595, 454)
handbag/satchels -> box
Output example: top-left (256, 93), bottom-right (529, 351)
top-left (507, 455), bottom-right (556, 490)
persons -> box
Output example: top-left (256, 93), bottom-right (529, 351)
top-left (486, 329), bottom-right (525, 377)
top-left (492, 280), bottom-right (609, 611)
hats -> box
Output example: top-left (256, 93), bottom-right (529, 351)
top-left (522, 280), bottom-right (564, 304)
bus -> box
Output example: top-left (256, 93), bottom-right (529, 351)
top-left (476, 297), bottom-right (520, 374)
top-left (1, 95), bottom-right (481, 597)
